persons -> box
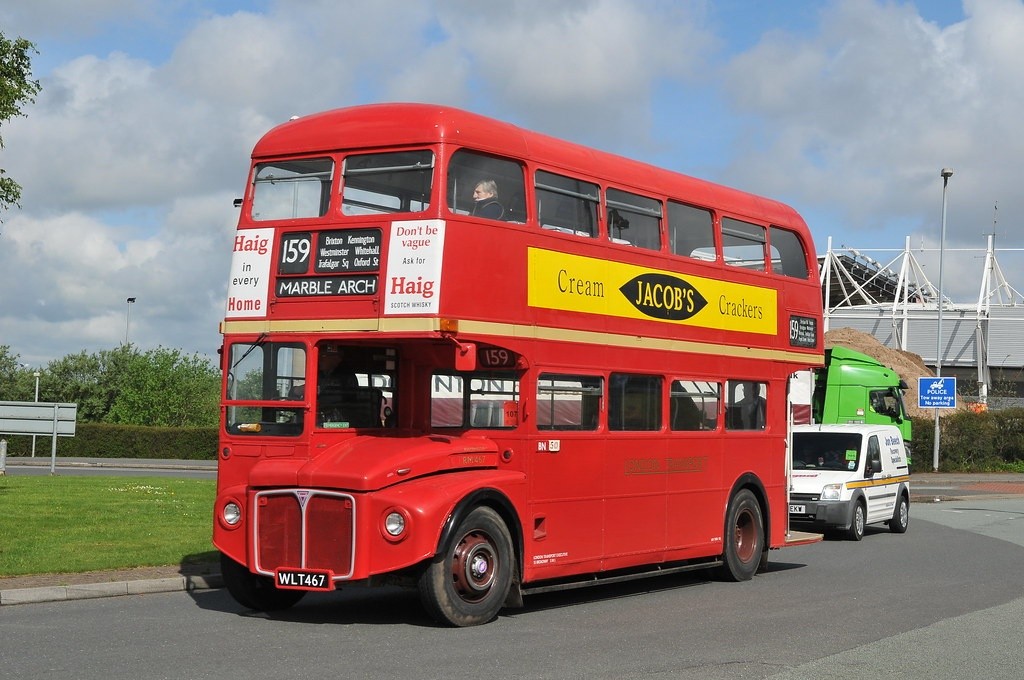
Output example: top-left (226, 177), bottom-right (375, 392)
top-left (468, 179), bottom-right (507, 222)
top-left (724, 381), bottom-right (766, 430)
top-left (294, 349), bottom-right (358, 416)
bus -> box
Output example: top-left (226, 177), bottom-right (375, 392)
top-left (211, 102), bottom-right (828, 629)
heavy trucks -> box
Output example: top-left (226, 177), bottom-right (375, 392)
top-left (282, 339), bottom-right (917, 483)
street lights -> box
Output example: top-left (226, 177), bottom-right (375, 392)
top-left (30, 372), bottom-right (41, 458)
top-left (931, 168), bottom-right (954, 472)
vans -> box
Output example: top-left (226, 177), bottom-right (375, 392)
top-left (785, 422), bottom-right (912, 541)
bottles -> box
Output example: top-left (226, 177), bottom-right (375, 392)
top-left (238, 424), bottom-right (261, 432)
